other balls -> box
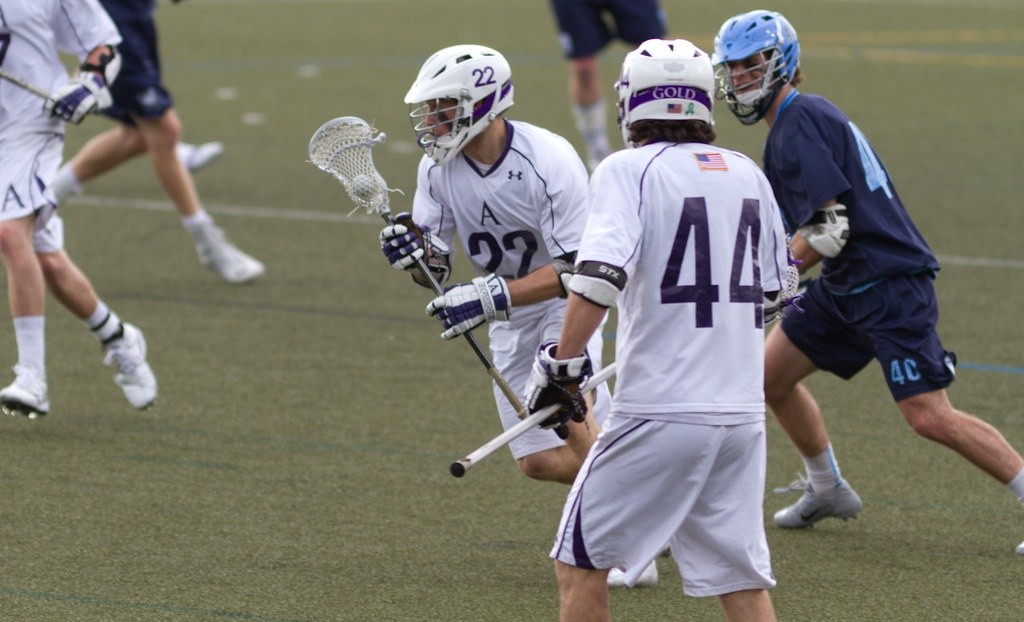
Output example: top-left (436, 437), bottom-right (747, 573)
top-left (352, 176), bottom-right (376, 200)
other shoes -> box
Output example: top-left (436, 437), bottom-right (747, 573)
top-left (607, 560), bottom-right (659, 588)
top-left (179, 141), bottom-right (223, 172)
top-left (197, 229), bottom-right (266, 284)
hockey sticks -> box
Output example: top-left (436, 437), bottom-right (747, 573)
top-left (305, 112), bottom-right (526, 416)
top-left (449, 235), bottom-right (800, 478)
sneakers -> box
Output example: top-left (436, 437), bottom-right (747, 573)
top-left (1016, 541), bottom-right (1024, 556)
top-left (0, 364), bottom-right (50, 419)
top-left (101, 321), bottom-right (158, 410)
top-left (773, 472), bottom-right (863, 529)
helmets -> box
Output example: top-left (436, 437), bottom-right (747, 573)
top-left (404, 44), bottom-right (515, 168)
top-left (710, 9), bottom-right (800, 126)
top-left (613, 39), bottom-right (716, 148)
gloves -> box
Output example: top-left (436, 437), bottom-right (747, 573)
top-left (379, 212), bottom-right (449, 270)
top-left (42, 72), bottom-right (113, 126)
top-left (522, 338), bottom-right (594, 440)
top-left (425, 272), bottom-right (512, 340)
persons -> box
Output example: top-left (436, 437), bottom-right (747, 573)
top-left (0, 0), bottom-right (265, 419)
top-left (378, 12), bottom-right (1024, 622)
top-left (549, 0), bottom-right (670, 172)
